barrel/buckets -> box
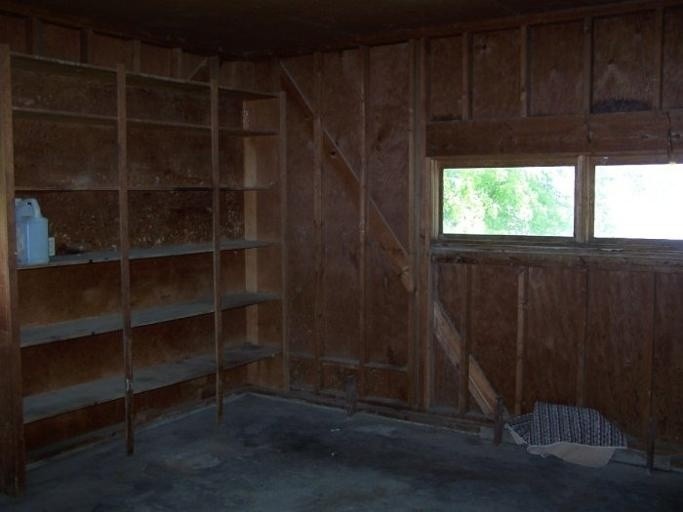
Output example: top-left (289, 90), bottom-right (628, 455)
top-left (15, 197), bottom-right (49, 266)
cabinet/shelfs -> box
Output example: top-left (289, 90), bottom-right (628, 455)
top-left (0, 50), bottom-right (289, 496)
